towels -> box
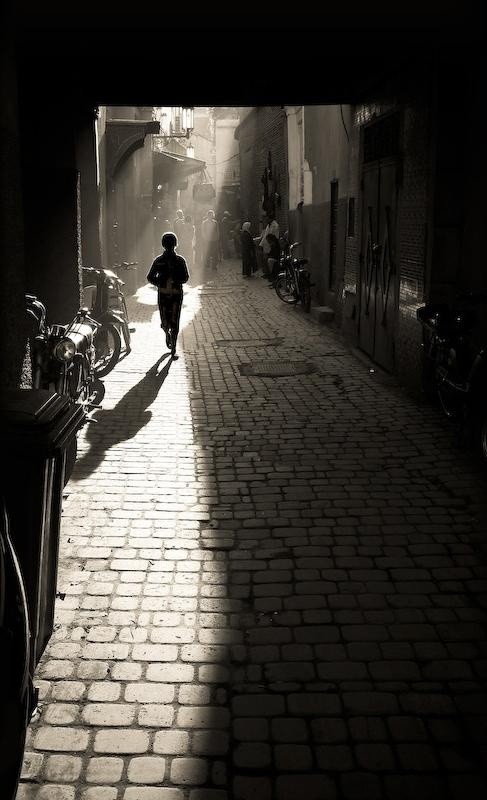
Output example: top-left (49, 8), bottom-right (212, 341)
top-left (0, 387), bottom-right (86, 669)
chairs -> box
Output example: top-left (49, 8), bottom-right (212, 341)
top-left (166, 332), bottom-right (178, 360)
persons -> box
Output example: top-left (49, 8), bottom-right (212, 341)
top-left (146, 232), bottom-right (189, 356)
top-left (173, 209), bottom-right (279, 277)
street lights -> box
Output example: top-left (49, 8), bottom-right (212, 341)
top-left (191, 225), bottom-right (198, 247)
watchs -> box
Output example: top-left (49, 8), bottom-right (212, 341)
top-left (24, 261), bottom-right (139, 428)
top-left (416, 304), bottom-right (487, 423)
top-left (272, 240), bottom-right (316, 313)
